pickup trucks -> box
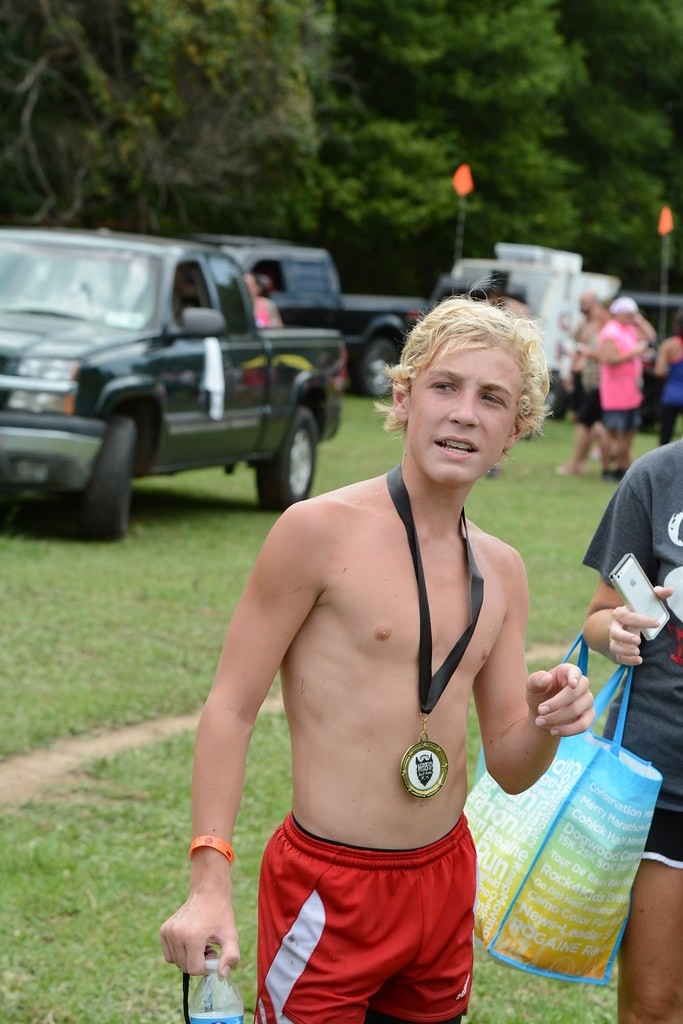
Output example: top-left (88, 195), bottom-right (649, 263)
top-left (196, 231), bottom-right (432, 400)
top-left (0, 221), bottom-right (347, 540)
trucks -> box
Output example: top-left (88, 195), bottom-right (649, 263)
top-left (436, 244), bottom-right (622, 421)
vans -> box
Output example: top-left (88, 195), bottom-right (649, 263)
top-left (615, 290), bottom-right (683, 383)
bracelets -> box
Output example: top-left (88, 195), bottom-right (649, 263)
top-left (189, 836), bottom-right (233, 867)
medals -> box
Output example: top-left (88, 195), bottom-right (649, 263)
top-left (400, 733), bottom-right (450, 799)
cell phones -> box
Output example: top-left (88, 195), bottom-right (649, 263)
top-left (609, 553), bottom-right (670, 641)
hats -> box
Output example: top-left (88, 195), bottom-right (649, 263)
top-left (610, 296), bottom-right (638, 316)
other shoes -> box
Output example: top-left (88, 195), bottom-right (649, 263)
top-left (555, 463), bottom-right (582, 475)
top-left (602, 468), bottom-right (628, 484)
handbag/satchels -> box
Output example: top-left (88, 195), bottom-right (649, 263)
top-left (462, 629), bottom-right (666, 987)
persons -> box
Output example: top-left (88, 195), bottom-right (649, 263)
top-left (554, 290), bottom-right (683, 481)
top-left (158, 296), bottom-right (596, 1024)
top-left (241, 273), bottom-right (282, 329)
top-left (582, 437), bottom-right (682, 1024)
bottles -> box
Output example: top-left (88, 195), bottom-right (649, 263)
top-left (188, 958), bottom-right (246, 1024)
